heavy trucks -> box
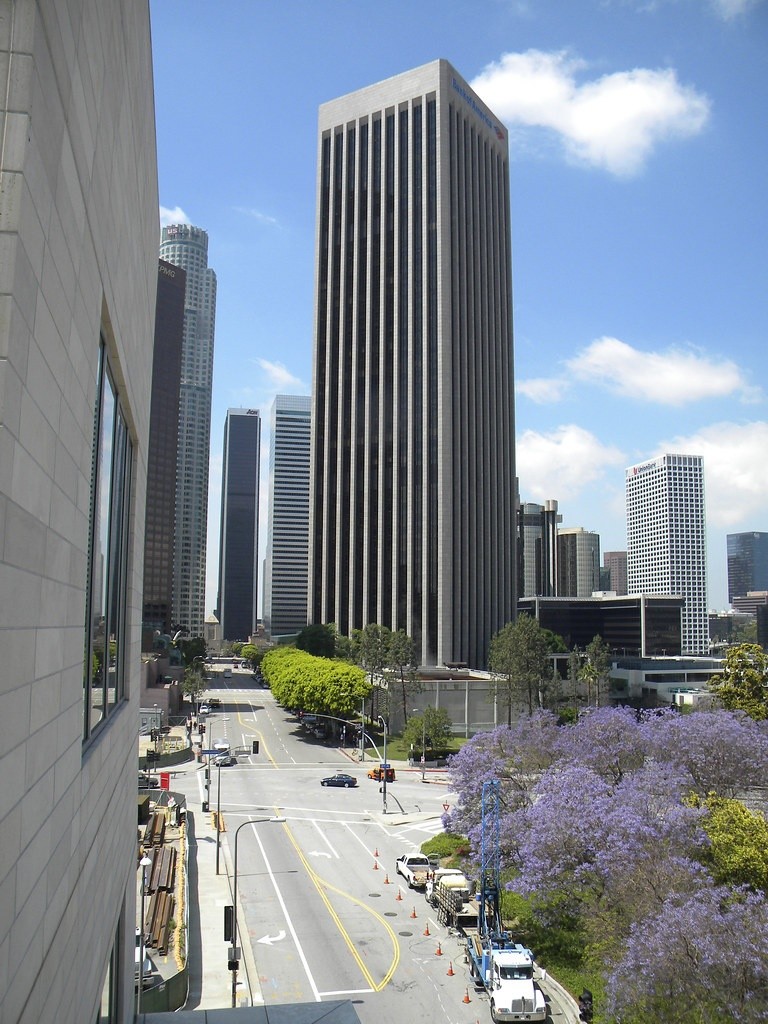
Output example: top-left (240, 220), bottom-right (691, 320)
top-left (425, 867), bottom-right (495, 930)
top-left (464, 934), bottom-right (549, 1024)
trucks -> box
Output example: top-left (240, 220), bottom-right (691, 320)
top-left (212, 737), bottom-right (232, 767)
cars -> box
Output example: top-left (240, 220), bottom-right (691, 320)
top-left (367, 766), bottom-right (384, 781)
top-left (138, 772), bottom-right (158, 790)
top-left (223, 660), bottom-right (272, 690)
top-left (320, 773), bottom-right (358, 789)
top-left (131, 927), bottom-right (155, 988)
top-left (290, 706), bottom-right (327, 740)
top-left (197, 698), bottom-right (222, 714)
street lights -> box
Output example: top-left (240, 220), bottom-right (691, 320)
top-left (340, 693), bottom-right (365, 762)
top-left (377, 715), bottom-right (387, 814)
top-left (216, 753), bottom-right (250, 875)
top-left (230, 817), bottom-right (287, 1006)
top-left (135, 850), bottom-right (153, 1015)
top-left (207, 718), bottom-right (231, 801)
top-left (195, 677), bottom-right (213, 732)
top-left (413, 708), bottom-right (426, 780)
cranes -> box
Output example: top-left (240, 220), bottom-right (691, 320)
top-left (469, 779), bottom-right (516, 960)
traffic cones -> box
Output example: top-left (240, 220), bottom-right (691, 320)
top-left (383, 873), bottom-right (390, 884)
top-left (409, 905), bottom-right (418, 918)
top-left (461, 987), bottom-right (472, 1003)
top-left (421, 922), bottom-right (431, 936)
top-left (394, 889), bottom-right (403, 900)
top-left (446, 960), bottom-right (456, 976)
top-left (433, 941), bottom-right (444, 956)
top-left (372, 848), bottom-right (380, 857)
top-left (372, 860), bottom-right (380, 871)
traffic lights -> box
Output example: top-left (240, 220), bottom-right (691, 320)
top-left (298, 710), bottom-right (304, 720)
top-left (151, 728), bottom-right (157, 742)
top-left (146, 749), bottom-right (155, 762)
top-left (386, 768), bottom-right (396, 782)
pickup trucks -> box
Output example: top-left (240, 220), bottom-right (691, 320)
top-left (395, 853), bottom-right (439, 889)
